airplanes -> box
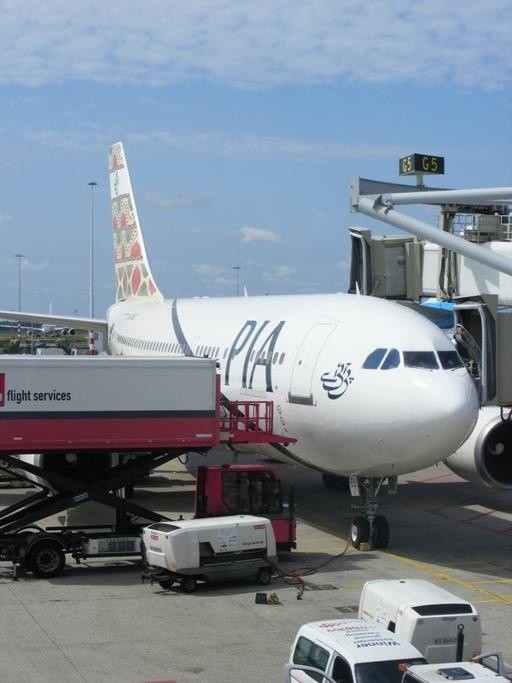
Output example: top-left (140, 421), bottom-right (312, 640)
top-left (0, 138), bottom-right (512, 552)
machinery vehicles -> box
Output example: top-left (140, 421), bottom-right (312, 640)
top-left (0, 354), bottom-right (301, 578)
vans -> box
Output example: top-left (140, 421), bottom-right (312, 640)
top-left (283, 617), bottom-right (504, 683)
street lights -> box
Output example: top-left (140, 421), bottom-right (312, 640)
top-left (233, 264), bottom-right (241, 296)
top-left (13, 254), bottom-right (26, 314)
top-left (85, 181), bottom-right (101, 319)
